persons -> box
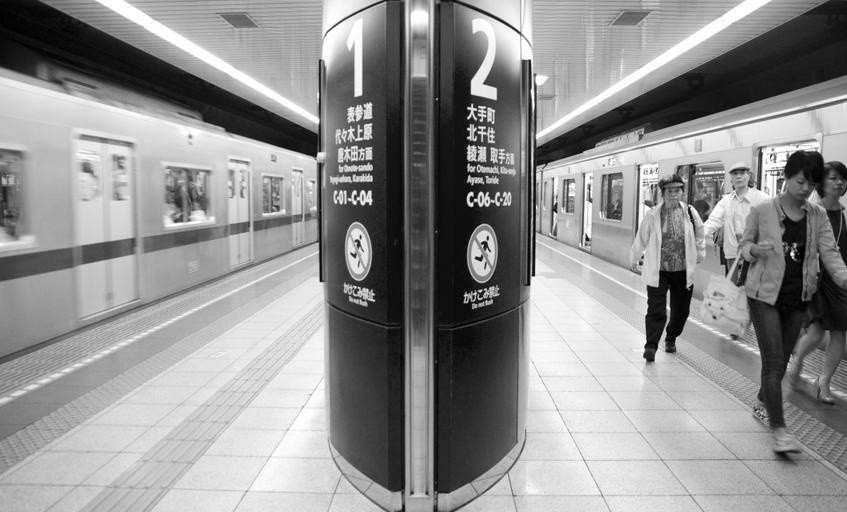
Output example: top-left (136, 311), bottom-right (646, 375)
top-left (739, 151), bottom-right (845, 454)
top-left (168, 169), bottom-right (210, 224)
top-left (714, 172), bottom-right (758, 279)
top-left (629, 173), bottom-right (708, 362)
top-left (783, 161), bottom-right (846, 404)
top-left (552, 192), bottom-right (559, 237)
top-left (701, 163), bottom-right (774, 341)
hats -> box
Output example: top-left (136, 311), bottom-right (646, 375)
top-left (659, 175), bottom-right (684, 190)
top-left (729, 163), bottom-right (749, 173)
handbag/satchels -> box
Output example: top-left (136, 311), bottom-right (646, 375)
top-left (700, 275), bottom-right (752, 328)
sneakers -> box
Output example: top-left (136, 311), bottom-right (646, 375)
top-left (644, 352), bottom-right (654, 361)
top-left (773, 437), bottom-right (802, 454)
top-left (665, 340), bottom-right (675, 352)
top-left (752, 403), bottom-right (768, 425)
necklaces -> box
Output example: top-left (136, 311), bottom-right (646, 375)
top-left (821, 200), bottom-right (843, 251)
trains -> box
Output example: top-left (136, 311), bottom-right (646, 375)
top-left (531, 71), bottom-right (847, 367)
top-left (0, 64), bottom-right (321, 367)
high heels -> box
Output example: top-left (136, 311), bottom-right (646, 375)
top-left (814, 377), bottom-right (836, 404)
